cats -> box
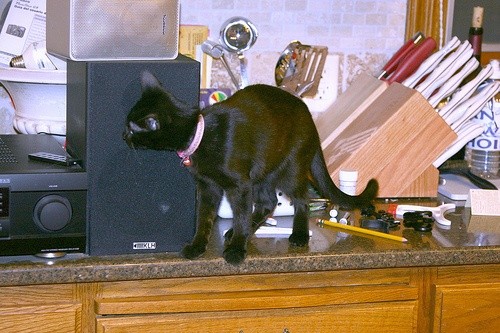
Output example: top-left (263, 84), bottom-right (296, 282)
top-left (121, 71), bottom-right (379, 264)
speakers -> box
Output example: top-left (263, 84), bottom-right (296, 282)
top-left (66, 53), bottom-right (200, 257)
top-left (46, 0), bottom-right (180, 62)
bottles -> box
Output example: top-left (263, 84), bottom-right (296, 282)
top-left (459, 27), bottom-right (484, 89)
top-left (464, 59), bottom-right (500, 178)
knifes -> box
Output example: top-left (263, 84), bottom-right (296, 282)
top-left (374, 31), bottom-right (500, 169)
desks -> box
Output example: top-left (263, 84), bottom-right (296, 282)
top-left (0, 215), bottom-right (500, 333)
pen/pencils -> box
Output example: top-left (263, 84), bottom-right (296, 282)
top-left (315, 218), bottom-right (408, 243)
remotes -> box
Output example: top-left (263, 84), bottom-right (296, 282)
top-left (29, 152), bottom-right (81, 166)
